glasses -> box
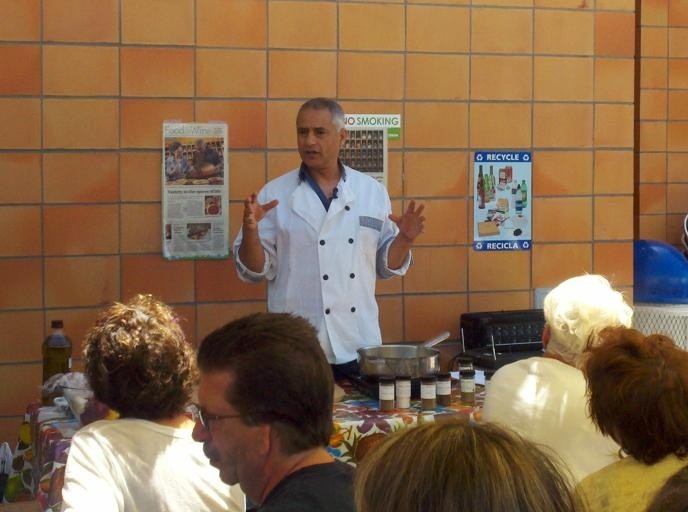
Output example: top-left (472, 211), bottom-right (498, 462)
top-left (190, 402), bottom-right (269, 433)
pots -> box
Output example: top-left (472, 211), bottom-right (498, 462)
top-left (354, 330), bottom-right (453, 378)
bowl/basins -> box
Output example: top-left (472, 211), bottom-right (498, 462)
top-left (57, 383), bottom-right (117, 424)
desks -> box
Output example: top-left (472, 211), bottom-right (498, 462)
top-left (0, 369), bottom-right (499, 512)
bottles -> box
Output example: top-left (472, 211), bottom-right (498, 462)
top-left (378, 378), bottom-right (395, 412)
top-left (475, 164), bottom-right (529, 215)
top-left (420, 375), bottom-right (436, 408)
top-left (458, 357), bottom-right (472, 372)
top-left (457, 370), bottom-right (476, 405)
top-left (41, 321), bottom-right (73, 403)
top-left (396, 375), bottom-right (410, 410)
top-left (434, 371), bottom-right (451, 405)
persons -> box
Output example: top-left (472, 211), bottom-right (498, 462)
top-left (481, 266), bottom-right (633, 492)
top-left (571, 324), bottom-right (688, 512)
top-left (192, 311), bottom-right (361, 512)
top-left (232, 98), bottom-right (426, 382)
top-left (352, 416), bottom-right (593, 512)
top-left (60, 294), bottom-right (246, 512)
top-left (646, 463), bottom-right (688, 512)
